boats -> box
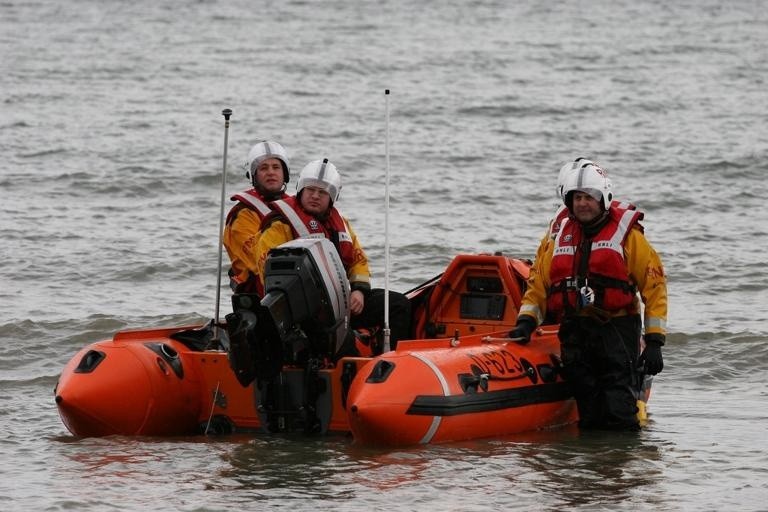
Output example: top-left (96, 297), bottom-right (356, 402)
top-left (53, 89), bottom-right (653, 449)
top-left (55, 252), bottom-right (652, 447)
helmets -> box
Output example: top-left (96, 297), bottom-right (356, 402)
top-left (295, 158), bottom-right (343, 206)
top-left (557, 157), bottom-right (596, 202)
top-left (561, 163), bottom-right (613, 212)
top-left (245, 141), bottom-right (291, 185)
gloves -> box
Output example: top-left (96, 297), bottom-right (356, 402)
top-left (508, 322), bottom-right (532, 345)
top-left (638, 343), bottom-right (664, 376)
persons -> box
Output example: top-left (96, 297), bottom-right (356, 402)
top-left (535, 158), bottom-right (654, 429)
top-left (258, 159), bottom-right (416, 356)
top-left (223, 140), bottom-right (290, 298)
top-left (508, 161), bottom-right (668, 433)
